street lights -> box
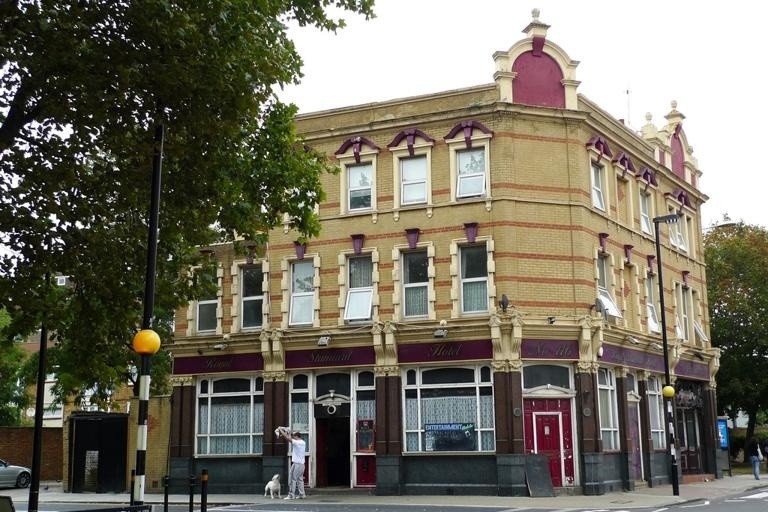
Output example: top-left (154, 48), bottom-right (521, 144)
top-left (134, 326), bottom-right (164, 510)
top-left (651, 213), bottom-right (682, 488)
top-left (664, 384), bottom-right (680, 496)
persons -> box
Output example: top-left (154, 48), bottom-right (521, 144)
top-left (277, 426), bottom-right (307, 500)
top-left (747, 436), bottom-right (760, 480)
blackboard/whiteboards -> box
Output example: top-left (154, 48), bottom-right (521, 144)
top-left (526, 454), bottom-right (554, 496)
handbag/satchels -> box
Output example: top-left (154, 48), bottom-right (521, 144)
top-left (756, 444), bottom-right (762, 461)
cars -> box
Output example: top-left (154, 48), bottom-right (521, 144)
top-left (0, 457), bottom-right (31, 488)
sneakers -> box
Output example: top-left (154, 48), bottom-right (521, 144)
top-left (284, 497), bottom-right (294, 500)
top-left (296, 496), bottom-right (305, 499)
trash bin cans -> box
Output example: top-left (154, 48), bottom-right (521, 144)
top-left (375, 455), bottom-right (401, 496)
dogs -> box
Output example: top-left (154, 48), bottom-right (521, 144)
top-left (264, 473), bottom-right (282, 500)
